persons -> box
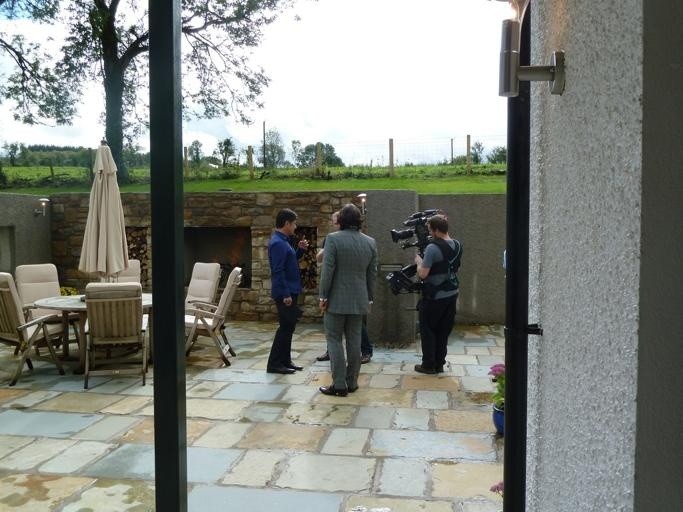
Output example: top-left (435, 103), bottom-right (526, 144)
top-left (264, 209), bottom-right (309, 375)
top-left (317, 211), bottom-right (373, 365)
top-left (412, 210), bottom-right (462, 373)
top-left (318, 203), bottom-right (379, 398)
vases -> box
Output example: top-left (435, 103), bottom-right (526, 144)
top-left (492, 400), bottom-right (505, 434)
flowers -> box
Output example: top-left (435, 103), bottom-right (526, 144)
top-left (487, 363), bottom-right (506, 410)
top-left (60, 286), bottom-right (80, 296)
top-left (488, 482), bottom-right (504, 499)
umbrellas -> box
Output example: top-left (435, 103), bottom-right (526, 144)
top-left (76, 137), bottom-right (140, 282)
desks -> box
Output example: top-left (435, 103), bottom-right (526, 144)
top-left (34, 293), bottom-right (153, 360)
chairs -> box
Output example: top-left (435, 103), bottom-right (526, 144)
top-left (14, 262), bottom-right (81, 359)
top-left (184, 261), bottom-right (223, 315)
top-left (83, 282), bottom-right (151, 389)
top-left (0, 271), bottom-right (66, 386)
top-left (100, 259), bottom-right (141, 283)
top-left (184, 266), bottom-right (244, 366)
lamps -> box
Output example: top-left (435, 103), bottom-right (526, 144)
top-left (356, 193), bottom-right (367, 215)
top-left (498, 19), bottom-right (565, 97)
top-left (34, 198), bottom-right (50, 217)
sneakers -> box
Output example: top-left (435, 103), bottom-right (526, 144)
top-left (414, 363), bottom-right (444, 374)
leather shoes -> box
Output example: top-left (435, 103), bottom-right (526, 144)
top-left (359, 350), bottom-right (373, 364)
top-left (282, 361), bottom-right (304, 370)
top-left (318, 385), bottom-right (348, 397)
top-left (316, 353), bottom-right (330, 361)
top-left (266, 364), bottom-right (297, 374)
